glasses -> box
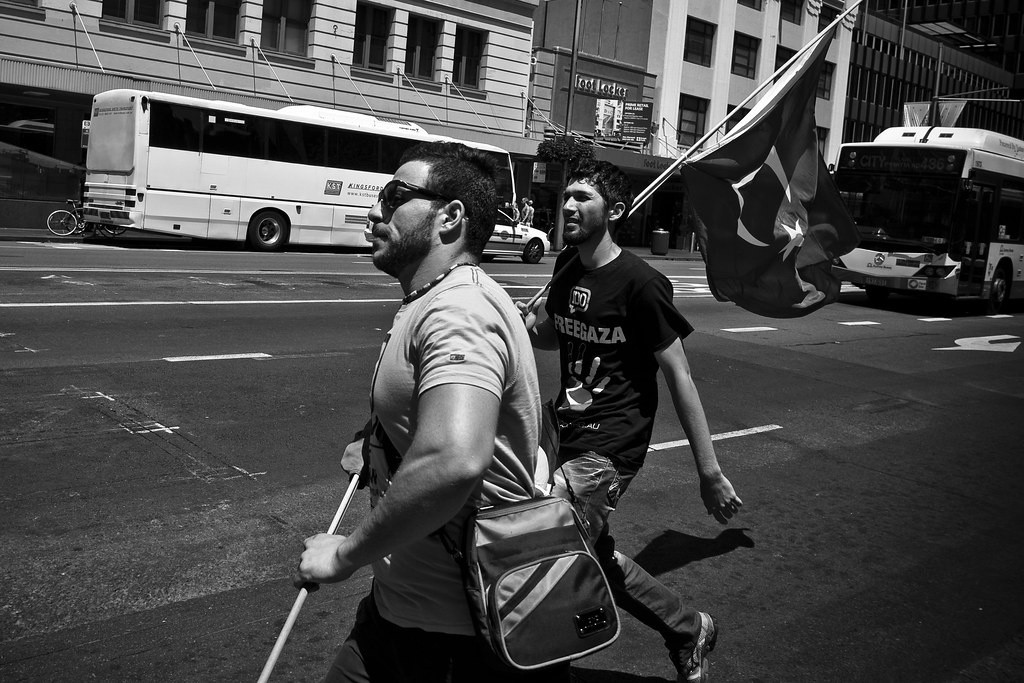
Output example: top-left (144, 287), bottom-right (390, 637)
top-left (378, 179), bottom-right (473, 219)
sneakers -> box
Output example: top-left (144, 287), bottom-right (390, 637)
top-left (668, 612), bottom-right (719, 683)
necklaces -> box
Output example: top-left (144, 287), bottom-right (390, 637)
top-left (401, 259), bottom-right (475, 305)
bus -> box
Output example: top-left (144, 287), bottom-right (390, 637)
top-left (81, 88), bottom-right (551, 264)
top-left (826, 126), bottom-right (1024, 314)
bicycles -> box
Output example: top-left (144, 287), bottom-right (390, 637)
top-left (46, 198), bottom-right (128, 236)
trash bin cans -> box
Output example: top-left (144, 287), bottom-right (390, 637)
top-left (651, 228), bottom-right (669, 255)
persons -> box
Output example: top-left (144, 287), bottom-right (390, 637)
top-left (293, 141), bottom-right (545, 683)
top-left (512, 197), bottom-right (534, 228)
top-left (77, 171), bottom-right (91, 221)
top-left (508, 160), bottom-right (743, 683)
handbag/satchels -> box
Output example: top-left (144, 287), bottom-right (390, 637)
top-left (457, 494), bottom-right (623, 672)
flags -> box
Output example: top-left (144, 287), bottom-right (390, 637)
top-left (677, 19), bottom-right (862, 319)
